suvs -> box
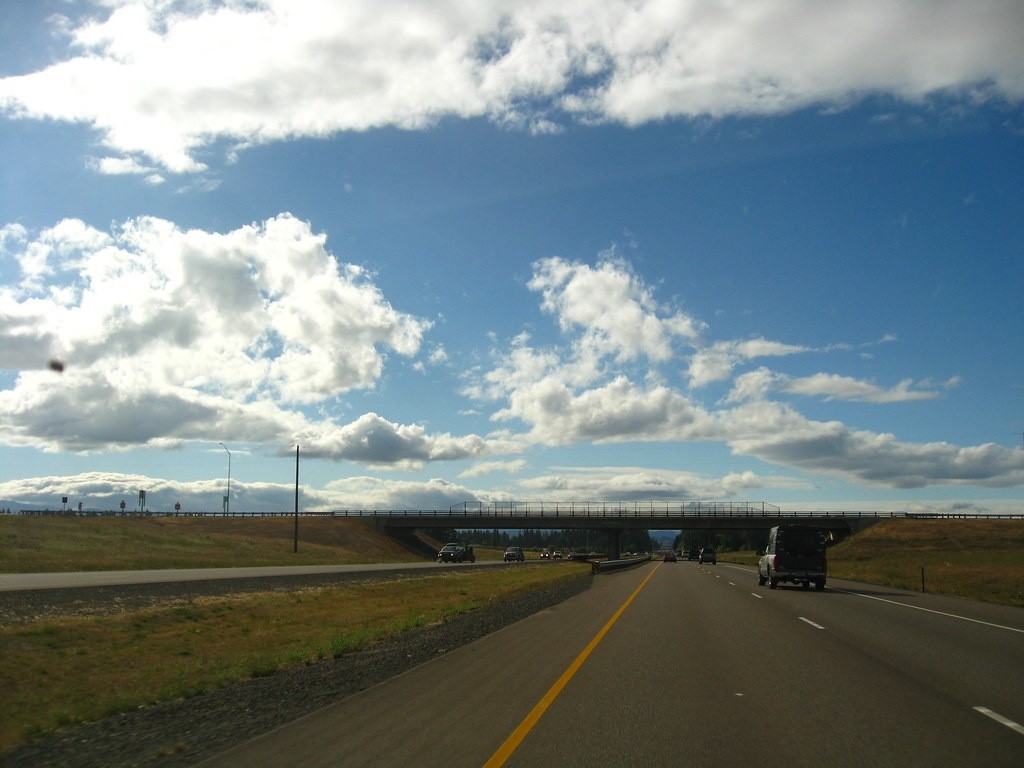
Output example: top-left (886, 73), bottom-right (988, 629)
top-left (698, 548), bottom-right (716, 565)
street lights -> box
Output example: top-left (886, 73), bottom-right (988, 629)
top-left (220, 443), bottom-right (230, 516)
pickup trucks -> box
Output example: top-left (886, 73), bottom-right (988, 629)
top-left (756, 525), bottom-right (828, 591)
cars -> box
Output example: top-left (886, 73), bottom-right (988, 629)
top-left (681, 550), bottom-right (690, 557)
top-left (568, 551), bottom-right (576, 560)
top-left (540, 550), bottom-right (550, 559)
top-left (438, 542), bottom-right (466, 564)
top-left (688, 549), bottom-right (701, 560)
top-left (664, 552), bottom-right (678, 561)
top-left (504, 546), bottom-right (525, 562)
top-left (553, 551), bottom-right (563, 559)
